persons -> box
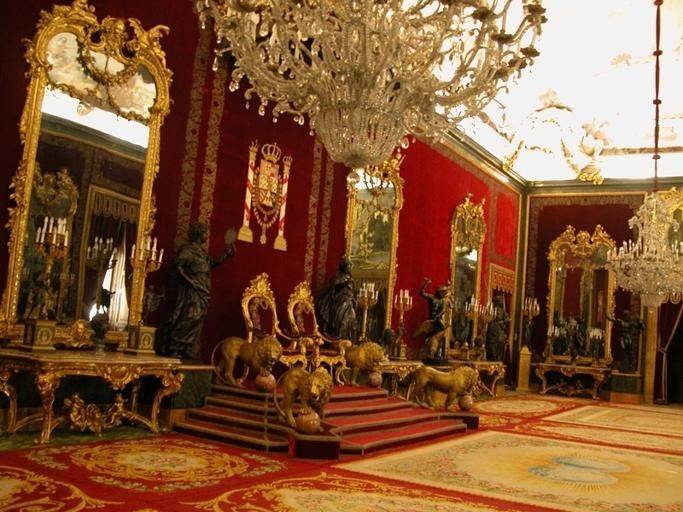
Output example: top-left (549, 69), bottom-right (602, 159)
top-left (164, 222), bottom-right (232, 359)
top-left (481, 296), bottom-right (509, 361)
top-left (318, 256), bottom-right (359, 340)
top-left (420, 278), bottom-right (448, 358)
top-left (606, 310), bottom-right (648, 370)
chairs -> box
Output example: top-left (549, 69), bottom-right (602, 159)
top-left (241, 272), bottom-right (350, 389)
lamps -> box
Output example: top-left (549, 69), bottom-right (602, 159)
top-left (197, 0), bottom-right (548, 169)
top-left (606, 0), bottom-right (681, 316)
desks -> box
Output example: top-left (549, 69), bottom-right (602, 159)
top-left (377, 360), bottom-right (424, 400)
top-left (536, 363), bottom-right (609, 400)
top-left (0, 348), bottom-right (182, 448)
top-left (453, 360), bottom-right (506, 400)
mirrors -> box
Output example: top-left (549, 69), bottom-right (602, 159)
top-left (4, 2), bottom-right (173, 357)
top-left (547, 224), bottom-right (615, 365)
top-left (447, 193), bottom-right (488, 357)
top-left (343, 162), bottom-right (405, 345)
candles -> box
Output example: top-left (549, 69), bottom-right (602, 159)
top-left (87, 237), bottom-right (116, 260)
top-left (131, 235), bottom-right (164, 261)
top-left (359, 283), bottom-right (378, 300)
top-left (395, 289), bottom-right (413, 305)
top-left (35, 215), bottom-right (68, 246)
